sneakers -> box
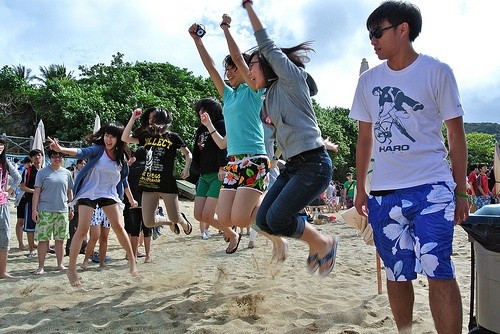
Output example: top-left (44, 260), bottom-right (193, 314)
top-left (202, 231), bottom-right (211, 240)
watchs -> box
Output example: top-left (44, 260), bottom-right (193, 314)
top-left (272, 156), bottom-right (279, 161)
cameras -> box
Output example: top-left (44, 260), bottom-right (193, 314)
top-left (193, 24), bottom-right (206, 38)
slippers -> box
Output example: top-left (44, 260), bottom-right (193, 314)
top-left (307, 253), bottom-right (319, 274)
top-left (181, 213), bottom-right (192, 234)
top-left (225, 226), bottom-right (235, 242)
top-left (171, 224), bottom-right (180, 234)
top-left (318, 235), bottom-right (337, 274)
top-left (226, 234), bottom-right (241, 254)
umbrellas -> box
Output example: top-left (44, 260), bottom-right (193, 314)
top-left (340, 57), bottom-right (382, 294)
top-left (32, 119), bottom-right (46, 170)
top-left (93, 115), bottom-right (101, 135)
top-left (494, 142), bottom-right (500, 197)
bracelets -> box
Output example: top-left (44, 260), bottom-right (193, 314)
top-left (243, 0), bottom-right (253, 8)
top-left (220, 22), bottom-right (230, 29)
top-left (455, 194), bottom-right (469, 198)
top-left (210, 130), bottom-right (217, 135)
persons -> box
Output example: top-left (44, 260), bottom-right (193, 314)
top-left (467, 164), bottom-right (493, 214)
top-left (242, 0), bottom-right (339, 278)
top-left (0, 136), bottom-right (22, 279)
top-left (349, 0), bottom-right (467, 334)
top-left (13, 147), bottom-right (155, 275)
top-left (344, 173), bottom-right (357, 209)
top-left (326, 181), bottom-right (341, 213)
top-left (121, 108), bottom-right (193, 235)
top-left (188, 13), bottom-right (289, 262)
top-left (48, 123), bottom-right (138, 287)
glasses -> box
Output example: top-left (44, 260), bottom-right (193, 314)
top-left (249, 61), bottom-right (259, 70)
top-left (225, 67), bottom-right (238, 72)
top-left (370, 26), bottom-right (394, 39)
top-left (52, 156), bottom-right (62, 159)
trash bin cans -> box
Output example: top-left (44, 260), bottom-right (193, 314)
top-left (461, 204), bottom-right (500, 326)
